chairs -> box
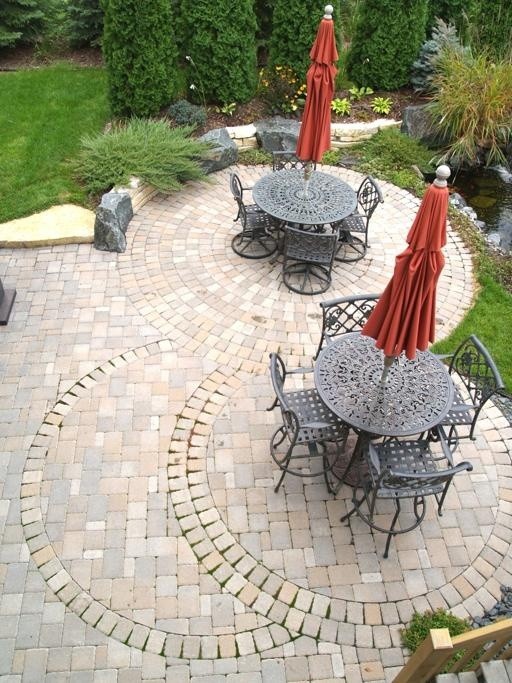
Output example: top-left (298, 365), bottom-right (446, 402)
top-left (339, 437), bottom-right (473, 563)
top-left (265, 350), bottom-right (350, 496)
top-left (313, 293), bottom-right (380, 362)
top-left (228, 147), bottom-right (384, 294)
top-left (414, 333), bottom-right (507, 447)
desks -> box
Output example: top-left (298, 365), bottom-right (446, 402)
top-left (314, 329), bottom-right (455, 489)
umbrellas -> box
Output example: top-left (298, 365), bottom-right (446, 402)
top-left (295, 5), bottom-right (341, 199)
top-left (360, 164), bottom-right (451, 381)
